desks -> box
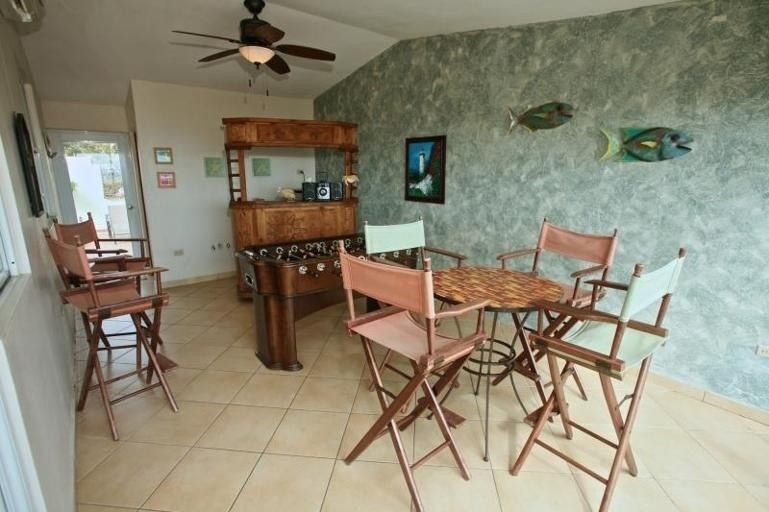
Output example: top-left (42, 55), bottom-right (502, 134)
top-left (426, 267), bottom-right (565, 428)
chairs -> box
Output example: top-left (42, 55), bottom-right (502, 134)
top-left (364, 215), bottom-right (468, 387)
top-left (41, 225), bottom-right (176, 442)
top-left (499, 215), bottom-right (617, 421)
top-left (51, 206), bottom-right (163, 347)
top-left (326, 241), bottom-right (490, 512)
top-left (527, 244), bottom-right (688, 512)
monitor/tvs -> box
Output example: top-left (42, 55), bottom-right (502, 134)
top-left (15, 113), bottom-right (43, 215)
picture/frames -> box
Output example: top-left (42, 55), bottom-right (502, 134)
top-left (154, 146), bottom-right (174, 165)
top-left (405, 133), bottom-right (447, 205)
top-left (14, 111), bottom-right (49, 219)
top-left (156, 171), bottom-right (176, 188)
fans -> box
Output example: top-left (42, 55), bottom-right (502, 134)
top-left (170, 0), bottom-right (335, 75)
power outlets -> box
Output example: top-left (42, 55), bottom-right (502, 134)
top-left (294, 167), bottom-right (305, 178)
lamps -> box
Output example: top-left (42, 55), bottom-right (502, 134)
top-left (238, 45), bottom-right (275, 70)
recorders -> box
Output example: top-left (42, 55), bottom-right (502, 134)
top-left (302, 181), bottom-right (343, 201)
